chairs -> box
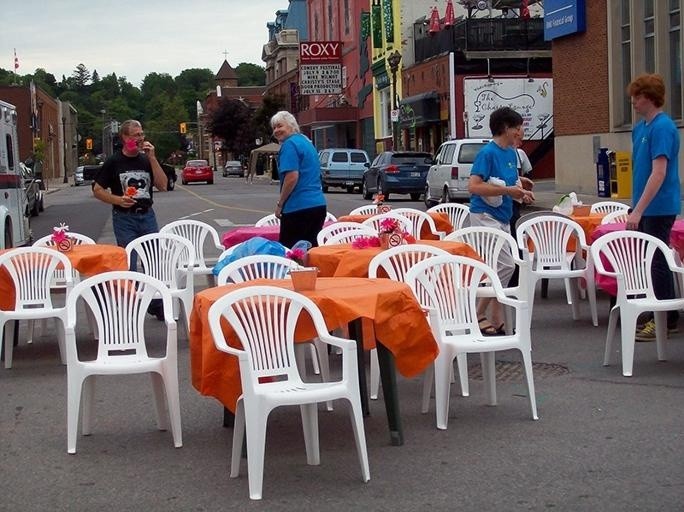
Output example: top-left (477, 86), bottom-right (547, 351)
top-left (120, 233), bottom-right (195, 352)
top-left (26, 232), bottom-right (97, 343)
top-left (516, 216), bottom-right (598, 327)
top-left (368, 243), bottom-right (471, 414)
top-left (404, 254), bottom-right (539, 430)
top-left (64, 270), bottom-right (183, 455)
top-left (217, 255), bottom-right (334, 411)
top-left (255, 203), bottom-right (470, 247)
top-left (1, 246), bottom-right (78, 369)
top-left (591, 200), bottom-right (630, 224)
top-left (591, 230), bottom-right (684, 378)
top-left (207, 285), bottom-right (370, 501)
top-left (159, 220), bottom-right (226, 322)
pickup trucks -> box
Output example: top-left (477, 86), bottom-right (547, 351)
top-left (82, 158), bottom-right (178, 194)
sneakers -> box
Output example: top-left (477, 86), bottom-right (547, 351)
top-left (636, 319), bottom-right (679, 342)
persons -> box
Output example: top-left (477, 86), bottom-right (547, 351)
top-left (89, 119), bottom-right (169, 325)
top-left (465, 104), bottom-right (536, 338)
top-left (507, 127), bottom-right (535, 287)
top-left (242, 157), bottom-right (250, 185)
top-left (622, 71), bottom-right (683, 344)
top-left (517, 0), bottom-right (530, 18)
top-left (268, 110), bottom-right (329, 251)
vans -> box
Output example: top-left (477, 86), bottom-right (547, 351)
top-left (422, 137), bottom-right (495, 208)
top-left (314, 148), bottom-right (371, 193)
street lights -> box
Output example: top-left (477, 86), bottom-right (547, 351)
top-left (385, 49), bottom-right (402, 149)
top-left (99, 109), bottom-right (106, 156)
top-left (61, 115), bottom-right (68, 183)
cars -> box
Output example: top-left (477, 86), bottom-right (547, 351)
top-left (360, 150), bottom-right (435, 201)
top-left (222, 160), bottom-right (244, 178)
top-left (19, 162), bottom-right (43, 216)
top-left (74, 164), bottom-right (86, 186)
top-left (180, 159), bottom-right (215, 186)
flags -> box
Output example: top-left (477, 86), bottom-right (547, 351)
top-left (12, 49), bottom-right (18, 69)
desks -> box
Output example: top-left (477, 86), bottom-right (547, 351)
top-left (588, 220), bottom-right (683, 327)
top-left (194, 277), bottom-right (408, 457)
top-left (223, 222), bottom-right (353, 249)
top-left (2, 244), bottom-right (127, 362)
top-left (307, 239), bottom-right (469, 353)
top-left (337, 212), bottom-right (454, 241)
top-left (528, 205), bottom-right (630, 298)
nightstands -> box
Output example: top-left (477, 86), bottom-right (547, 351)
top-left (515, 211), bottom-right (586, 304)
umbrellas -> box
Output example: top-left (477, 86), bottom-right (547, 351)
top-left (428, 5), bottom-right (440, 34)
top-left (442, 0), bottom-right (456, 30)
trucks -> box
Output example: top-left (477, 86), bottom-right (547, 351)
top-left (0, 98), bottom-right (33, 252)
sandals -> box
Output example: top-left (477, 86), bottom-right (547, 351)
top-left (478, 317), bottom-right (515, 336)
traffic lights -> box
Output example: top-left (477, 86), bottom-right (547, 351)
top-left (180, 123), bottom-right (186, 134)
top-left (86, 139), bottom-right (92, 150)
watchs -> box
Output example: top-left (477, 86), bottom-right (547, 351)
top-left (275, 199), bottom-right (283, 208)
